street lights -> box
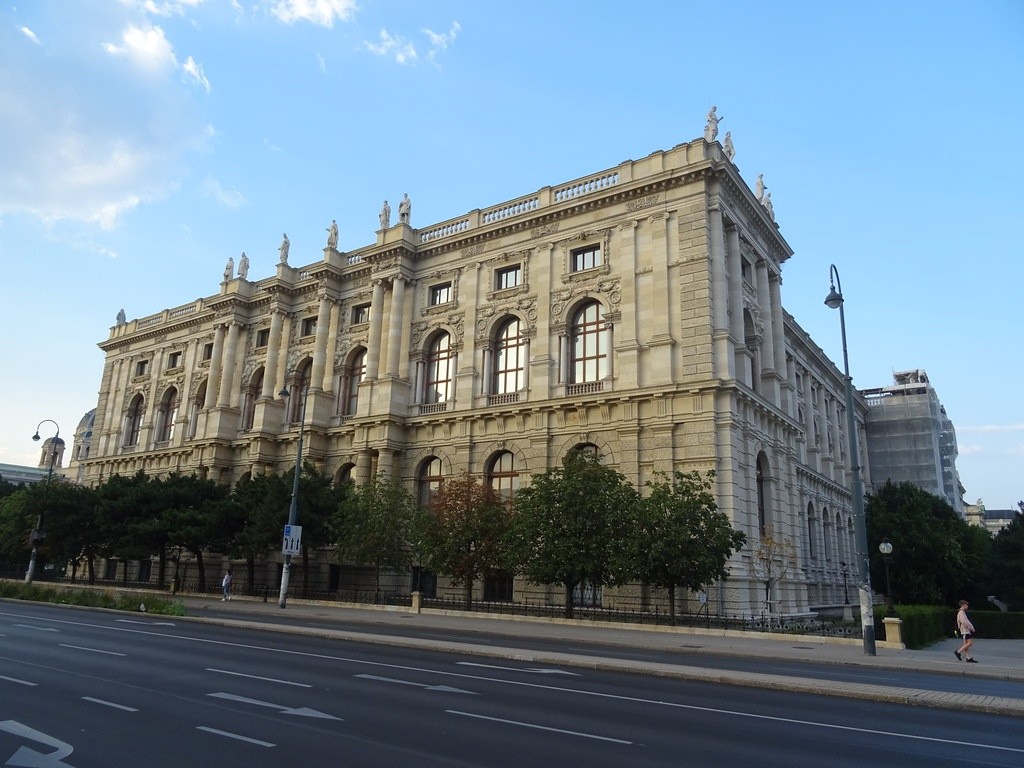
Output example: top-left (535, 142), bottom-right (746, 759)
top-left (276, 368), bottom-right (310, 608)
top-left (25, 418), bottom-right (60, 586)
top-left (823, 262), bottom-right (877, 659)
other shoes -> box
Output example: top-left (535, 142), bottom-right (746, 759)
top-left (221, 599), bottom-right (226, 602)
top-left (228, 597), bottom-right (231, 601)
top-left (966, 657), bottom-right (978, 663)
top-left (954, 651), bottom-right (962, 660)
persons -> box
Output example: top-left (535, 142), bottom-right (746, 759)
top-left (325, 220), bottom-right (338, 248)
top-left (223, 256), bottom-right (234, 281)
top-left (278, 233), bottom-right (290, 263)
top-left (704, 105), bottom-right (719, 143)
top-left (379, 200), bottom-right (391, 229)
top-left (954, 600), bottom-right (978, 662)
top-left (221, 569), bottom-right (233, 601)
top-left (724, 131), bottom-right (735, 163)
top-left (753, 172), bottom-right (774, 222)
top-left (116, 309), bottom-right (126, 325)
top-left (238, 252), bottom-right (249, 278)
top-left (398, 193), bottom-right (411, 224)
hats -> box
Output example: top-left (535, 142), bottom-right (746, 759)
top-left (959, 600), bottom-right (968, 606)
